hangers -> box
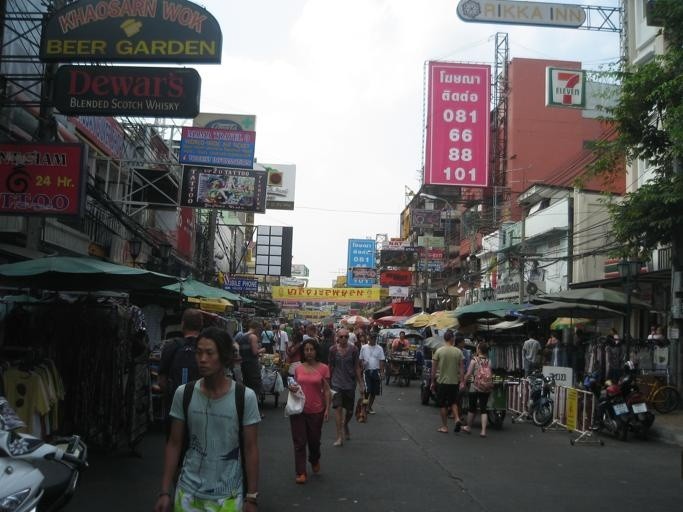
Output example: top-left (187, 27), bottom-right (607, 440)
top-left (16, 349), bottom-right (53, 379)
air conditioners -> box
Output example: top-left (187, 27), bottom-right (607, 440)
top-left (523, 279), bottom-right (546, 297)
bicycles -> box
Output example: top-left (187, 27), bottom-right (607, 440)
top-left (629, 373), bottom-right (679, 415)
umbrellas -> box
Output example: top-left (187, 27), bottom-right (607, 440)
top-left (0, 255), bottom-right (256, 311)
top-left (447, 284), bottom-right (654, 370)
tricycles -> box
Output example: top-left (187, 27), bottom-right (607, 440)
top-left (257, 355), bottom-right (281, 407)
top-left (374, 327), bottom-right (517, 429)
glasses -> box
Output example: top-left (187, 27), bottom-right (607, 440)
top-left (340, 335), bottom-right (349, 338)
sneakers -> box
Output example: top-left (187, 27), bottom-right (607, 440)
top-left (312, 460), bottom-right (320, 473)
top-left (334, 438), bottom-right (343, 447)
top-left (295, 473), bottom-right (306, 483)
top-left (343, 424), bottom-right (351, 440)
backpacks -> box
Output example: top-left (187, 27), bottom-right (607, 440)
top-left (172, 344), bottom-right (198, 384)
top-left (474, 356), bottom-right (492, 392)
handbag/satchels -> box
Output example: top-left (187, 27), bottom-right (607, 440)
top-left (284, 384), bottom-right (305, 415)
top-left (355, 398), bottom-right (369, 423)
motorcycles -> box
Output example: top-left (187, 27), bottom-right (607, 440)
top-left (616, 361), bottom-right (655, 436)
top-left (579, 370), bottom-right (630, 441)
top-left (524, 372), bottom-right (556, 426)
top-left (0, 396), bottom-right (90, 512)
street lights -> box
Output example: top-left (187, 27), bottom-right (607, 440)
top-left (615, 250), bottom-right (644, 370)
top-left (420, 190), bottom-right (478, 256)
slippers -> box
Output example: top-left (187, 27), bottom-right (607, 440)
top-left (437, 427), bottom-right (448, 433)
top-left (480, 431), bottom-right (487, 437)
top-left (454, 420), bottom-right (462, 432)
top-left (462, 426), bottom-right (470, 432)
